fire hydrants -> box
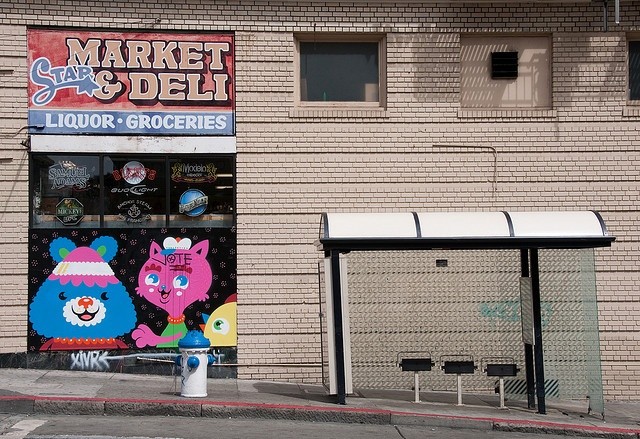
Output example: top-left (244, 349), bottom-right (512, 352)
top-left (174, 330), bottom-right (216, 398)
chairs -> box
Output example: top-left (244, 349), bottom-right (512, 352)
top-left (481, 356), bottom-right (520, 410)
top-left (440, 354), bottom-right (479, 406)
top-left (396, 349), bottom-right (436, 404)
